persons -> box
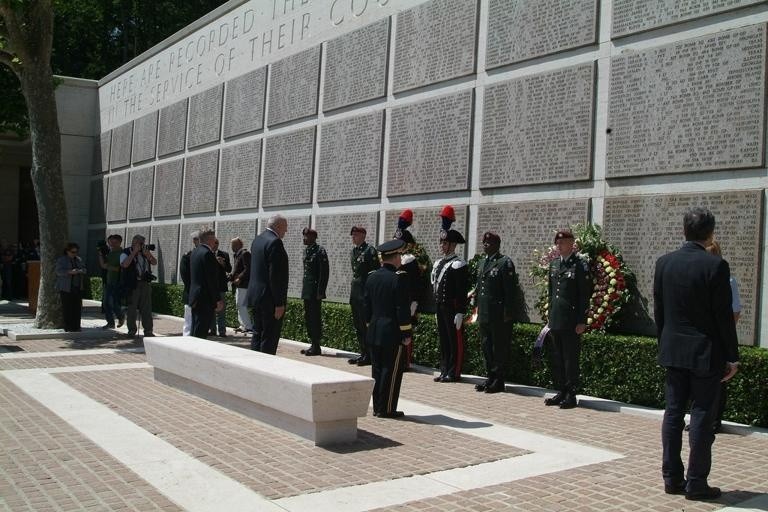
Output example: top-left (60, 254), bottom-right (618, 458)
top-left (98, 234), bottom-right (124, 331)
top-left (684, 242), bottom-right (742, 433)
top-left (653, 208), bottom-right (738, 501)
top-left (242, 215), bottom-right (289, 355)
top-left (363, 242), bottom-right (413, 418)
top-left (119, 235), bottom-right (157, 337)
top-left (474, 233), bottom-right (520, 393)
top-left (102, 235), bottom-right (113, 314)
top-left (393, 228), bottom-right (421, 373)
top-left (179, 228), bottom-right (231, 339)
top-left (544, 231), bottom-right (591, 409)
top-left (55, 242), bottom-right (87, 332)
top-left (301, 228), bottom-right (329, 356)
top-left (430, 229), bottom-right (469, 383)
top-left (226, 238), bottom-right (251, 332)
top-left (348, 226), bottom-right (381, 367)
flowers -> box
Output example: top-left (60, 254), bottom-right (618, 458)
top-left (378, 239), bottom-right (432, 278)
top-left (468, 251), bottom-right (503, 328)
top-left (530, 226), bottom-right (627, 333)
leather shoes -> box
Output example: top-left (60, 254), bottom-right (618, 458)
top-left (544, 395), bottom-right (565, 404)
top-left (349, 355), bottom-right (362, 363)
top-left (441, 375), bottom-right (455, 382)
top-left (686, 488), bottom-right (721, 499)
top-left (484, 385), bottom-right (504, 392)
top-left (475, 384), bottom-right (487, 390)
top-left (559, 398), bottom-right (575, 408)
top-left (359, 360), bottom-right (371, 366)
top-left (301, 349), bottom-right (321, 355)
top-left (434, 375), bottom-right (447, 382)
top-left (665, 482), bottom-right (685, 494)
top-left (377, 412), bottom-right (404, 417)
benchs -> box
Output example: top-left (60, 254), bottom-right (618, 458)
top-left (141, 336), bottom-right (376, 445)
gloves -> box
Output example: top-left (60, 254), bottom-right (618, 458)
top-left (410, 301), bottom-right (417, 316)
top-left (454, 313), bottom-right (465, 330)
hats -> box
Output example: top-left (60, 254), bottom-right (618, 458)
top-left (378, 240), bottom-right (406, 255)
top-left (485, 232), bottom-right (500, 240)
top-left (440, 229), bottom-right (465, 243)
top-left (393, 229), bottom-right (414, 243)
top-left (555, 232), bottom-right (574, 241)
top-left (351, 227), bottom-right (365, 232)
top-left (303, 228), bottom-right (317, 237)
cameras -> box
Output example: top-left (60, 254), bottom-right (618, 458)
top-left (144, 271), bottom-right (157, 283)
